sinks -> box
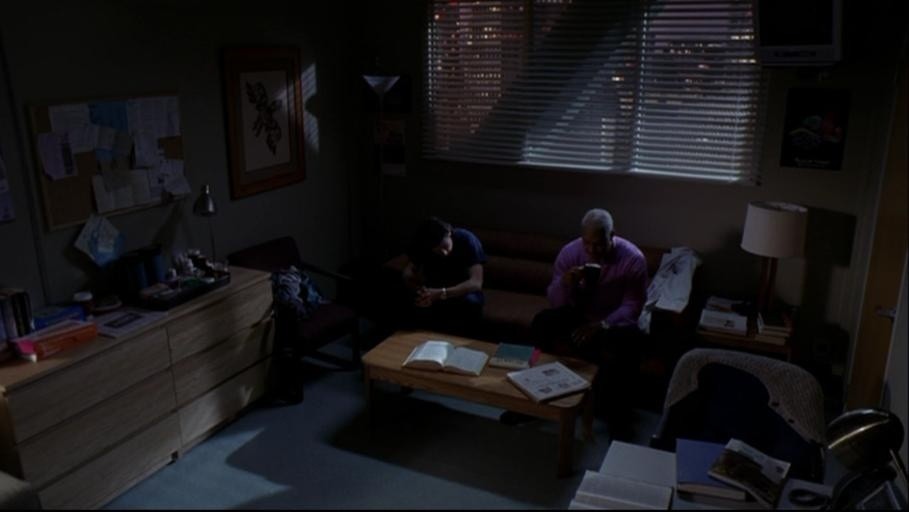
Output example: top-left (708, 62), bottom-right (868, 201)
top-left (388, 230), bottom-right (685, 410)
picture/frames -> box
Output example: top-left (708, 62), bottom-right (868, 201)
top-left (598, 439), bottom-right (833, 510)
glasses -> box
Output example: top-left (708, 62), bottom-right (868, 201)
top-left (193, 184), bottom-right (218, 263)
top-left (731, 200), bottom-right (808, 326)
top-left (825, 408), bottom-right (908, 489)
top-left (363, 75), bottom-right (400, 233)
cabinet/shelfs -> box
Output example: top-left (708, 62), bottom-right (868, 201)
top-left (1, 286), bottom-right (34, 343)
top-left (167, 247), bottom-right (214, 283)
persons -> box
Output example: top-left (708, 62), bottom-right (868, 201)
top-left (403, 219), bottom-right (483, 338)
top-left (531, 208), bottom-right (648, 372)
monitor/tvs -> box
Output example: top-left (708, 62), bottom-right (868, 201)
top-left (507, 360), bottom-right (592, 404)
top-left (675, 438), bottom-right (747, 500)
top-left (568, 469), bottom-right (675, 511)
top-left (696, 296), bottom-right (791, 346)
top-left (402, 340), bottom-right (491, 376)
top-left (0, 287), bottom-right (35, 340)
top-left (491, 343), bottom-right (535, 369)
top-left (708, 437), bottom-right (792, 510)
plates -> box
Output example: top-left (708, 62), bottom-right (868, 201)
top-left (0, 263), bottom-right (275, 509)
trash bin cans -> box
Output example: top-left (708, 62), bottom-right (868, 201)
top-left (600, 318), bottom-right (611, 332)
top-left (439, 287), bottom-right (450, 305)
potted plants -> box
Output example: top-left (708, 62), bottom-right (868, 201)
top-left (779, 87), bottom-right (855, 172)
top-left (220, 47), bottom-right (305, 199)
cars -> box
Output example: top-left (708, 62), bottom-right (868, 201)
top-left (210, 244), bottom-right (228, 279)
top-left (70, 290), bottom-right (93, 322)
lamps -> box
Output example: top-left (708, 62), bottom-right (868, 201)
top-left (649, 348), bottom-right (824, 484)
top-left (232, 235), bottom-right (361, 404)
